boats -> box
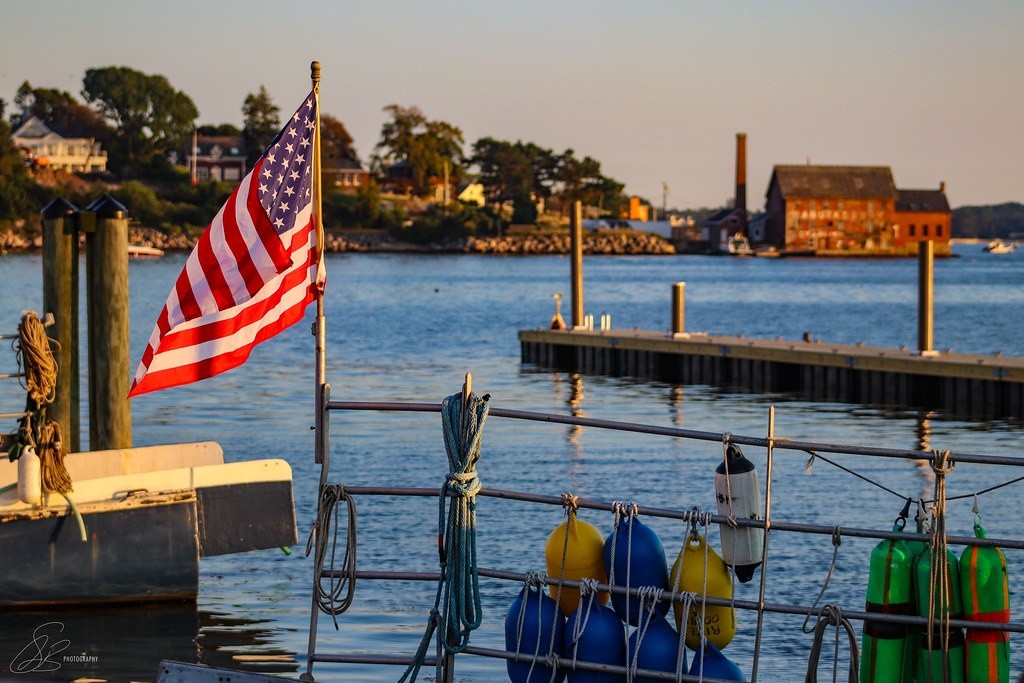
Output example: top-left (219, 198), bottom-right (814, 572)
top-left (718, 235), bottom-right (754, 257)
top-left (127, 246), bottom-right (165, 261)
top-left (982, 238), bottom-right (1017, 255)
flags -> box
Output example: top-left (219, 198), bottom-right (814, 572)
top-left (124, 81), bottom-right (328, 400)
